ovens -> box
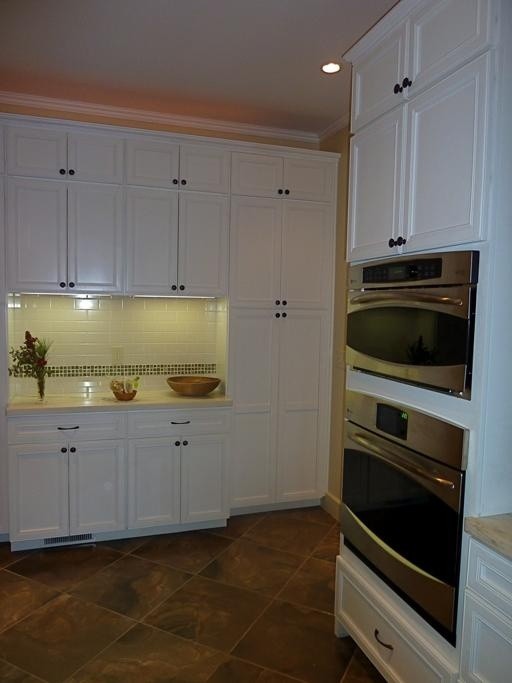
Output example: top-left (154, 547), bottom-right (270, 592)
top-left (339, 247), bottom-right (478, 651)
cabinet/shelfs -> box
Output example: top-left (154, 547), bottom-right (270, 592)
top-left (350, 1), bottom-right (512, 136)
top-left (3, 116), bottom-right (122, 302)
top-left (7, 411), bottom-right (127, 544)
top-left (333, 556), bottom-right (459, 683)
top-left (458, 534), bottom-right (512, 683)
top-left (217, 313), bottom-right (333, 519)
top-left (128, 407), bottom-right (230, 529)
top-left (344, 49), bottom-right (512, 266)
top-left (229, 138), bottom-right (336, 314)
top-left (120, 126), bottom-right (232, 305)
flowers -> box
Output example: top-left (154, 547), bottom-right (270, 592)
top-left (7, 330), bottom-right (55, 378)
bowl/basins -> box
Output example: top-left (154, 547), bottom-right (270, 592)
top-left (113, 390), bottom-right (137, 401)
top-left (166, 375), bottom-right (223, 396)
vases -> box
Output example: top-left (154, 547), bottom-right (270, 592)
top-left (37, 378), bottom-right (45, 401)
